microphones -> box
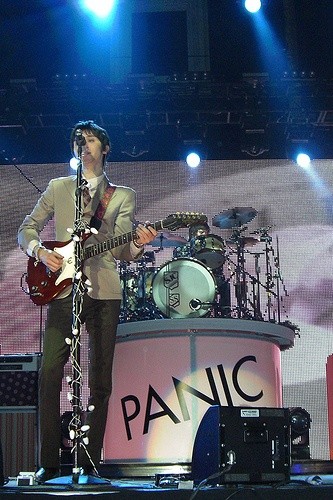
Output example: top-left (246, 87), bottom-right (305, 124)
top-left (75, 129), bottom-right (86, 146)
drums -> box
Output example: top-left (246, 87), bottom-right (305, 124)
top-left (190, 233), bottom-right (225, 272)
top-left (151, 257), bottom-right (219, 319)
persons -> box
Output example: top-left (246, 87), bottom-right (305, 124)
top-left (18, 121), bottom-right (159, 484)
top-left (188, 222), bottom-right (232, 319)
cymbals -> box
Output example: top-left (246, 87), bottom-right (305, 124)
top-left (223, 237), bottom-right (260, 248)
top-left (211, 207), bottom-right (259, 229)
top-left (145, 231), bottom-right (188, 248)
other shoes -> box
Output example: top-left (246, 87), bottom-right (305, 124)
top-left (79, 468), bottom-right (96, 478)
top-left (34, 468), bottom-right (60, 485)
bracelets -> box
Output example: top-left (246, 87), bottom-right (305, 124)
top-left (34, 246), bottom-right (46, 262)
top-left (134, 240), bottom-right (146, 248)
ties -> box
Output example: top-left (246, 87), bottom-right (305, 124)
top-left (81, 185), bottom-right (91, 210)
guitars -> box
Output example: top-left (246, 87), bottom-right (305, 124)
top-left (26, 210), bottom-right (207, 305)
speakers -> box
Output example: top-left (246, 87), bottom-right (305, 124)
top-left (190, 405), bottom-right (292, 486)
